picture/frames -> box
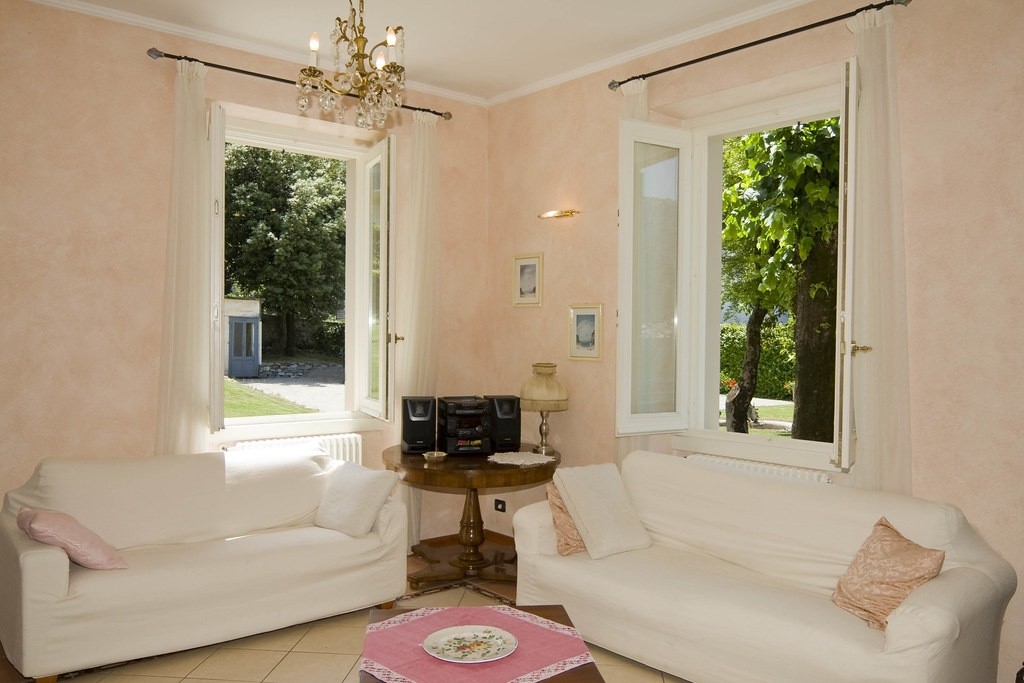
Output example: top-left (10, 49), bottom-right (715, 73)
top-left (567, 302), bottom-right (605, 364)
top-left (511, 252), bottom-right (544, 310)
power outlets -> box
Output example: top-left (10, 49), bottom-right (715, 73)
top-left (494, 499), bottom-right (506, 512)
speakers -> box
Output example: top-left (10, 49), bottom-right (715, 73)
top-left (401, 395), bottom-right (436, 454)
top-left (483, 394), bottom-right (523, 452)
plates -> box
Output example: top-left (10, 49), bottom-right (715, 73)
top-left (423, 625), bottom-right (519, 663)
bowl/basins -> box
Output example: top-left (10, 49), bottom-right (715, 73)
top-left (422, 452), bottom-right (447, 463)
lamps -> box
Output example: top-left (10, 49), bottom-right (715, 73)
top-left (519, 362), bottom-right (570, 456)
top-left (294, 0), bottom-right (409, 133)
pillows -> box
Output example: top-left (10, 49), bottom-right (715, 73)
top-left (545, 463), bottom-right (654, 560)
top-left (830, 515), bottom-right (946, 632)
top-left (16, 505), bottom-right (129, 571)
top-left (312, 462), bottom-right (400, 539)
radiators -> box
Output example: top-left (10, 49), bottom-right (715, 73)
top-left (685, 452), bottom-right (834, 486)
top-left (220, 433), bottom-right (364, 468)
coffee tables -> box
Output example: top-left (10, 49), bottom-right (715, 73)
top-left (358, 603), bottom-right (608, 683)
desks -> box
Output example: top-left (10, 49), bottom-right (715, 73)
top-left (381, 441), bottom-right (562, 590)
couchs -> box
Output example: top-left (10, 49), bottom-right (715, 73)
top-left (510, 447), bottom-right (1019, 683)
top-left (0, 444), bottom-right (410, 683)
